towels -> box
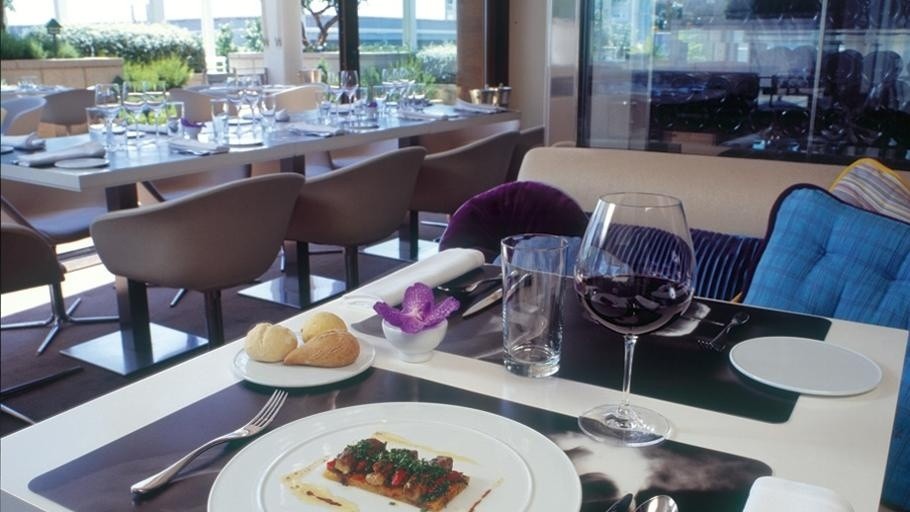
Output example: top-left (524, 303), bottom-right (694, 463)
top-left (435, 182), bottom-right (588, 254)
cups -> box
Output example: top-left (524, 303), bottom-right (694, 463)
top-left (211, 95), bottom-right (229, 144)
top-left (499, 233), bottom-right (570, 378)
top-left (165, 100), bottom-right (187, 139)
top-left (85, 106), bottom-right (104, 145)
top-left (313, 86), bottom-right (333, 126)
top-left (438, 81), bottom-right (514, 112)
top-left (353, 86), bottom-right (368, 120)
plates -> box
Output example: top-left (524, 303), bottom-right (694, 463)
top-left (206, 399), bottom-right (583, 512)
top-left (58, 158), bottom-right (110, 171)
top-left (233, 330), bottom-right (375, 388)
top-left (725, 334), bottom-right (884, 398)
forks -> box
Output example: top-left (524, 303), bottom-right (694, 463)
top-left (696, 309), bottom-right (750, 352)
top-left (129, 388), bottom-right (290, 495)
top-left (436, 270), bottom-right (520, 298)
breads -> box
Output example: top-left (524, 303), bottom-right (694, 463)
top-left (283, 329), bottom-right (360, 368)
top-left (301, 312), bottom-right (347, 342)
top-left (244, 322), bottom-right (298, 364)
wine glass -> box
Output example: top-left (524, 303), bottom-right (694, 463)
top-left (570, 191), bottom-right (699, 447)
top-left (94, 84), bottom-right (121, 152)
top-left (226, 75), bottom-right (280, 145)
top-left (186, 127), bottom-right (201, 140)
top-left (144, 82), bottom-right (167, 149)
top-left (329, 71), bottom-right (359, 125)
top-left (371, 67), bottom-right (425, 119)
top-left (122, 81), bottom-right (145, 151)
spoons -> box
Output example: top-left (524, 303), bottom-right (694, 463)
top-left (634, 494), bottom-right (679, 512)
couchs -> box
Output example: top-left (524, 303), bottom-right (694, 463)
top-left (437, 147), bottom-right (910, 302)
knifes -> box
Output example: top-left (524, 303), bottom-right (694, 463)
top-left (606, 493), bottom-right (633, 512)
top-left (463, 272), bottom-right (533, 318)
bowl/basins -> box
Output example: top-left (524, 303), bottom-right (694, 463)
top-left (383, 314), bottom-right (448, 363)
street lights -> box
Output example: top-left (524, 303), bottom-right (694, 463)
top-left (45, 17), bottom-right (63, 57)
